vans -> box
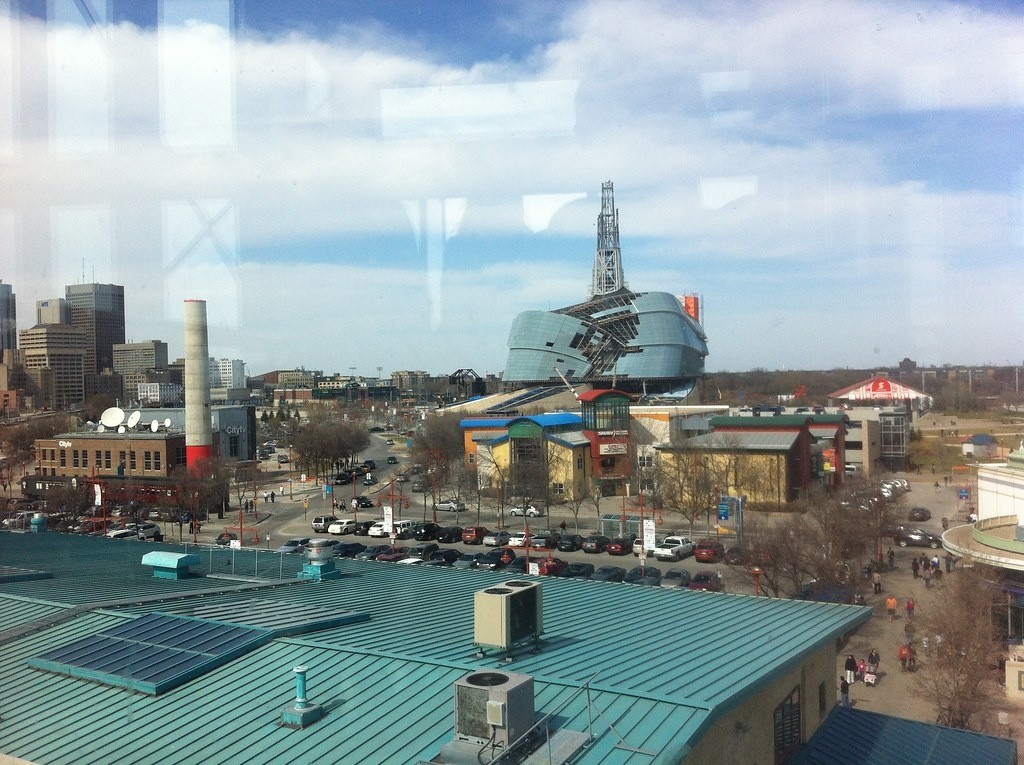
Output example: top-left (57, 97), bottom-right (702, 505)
top-left (632, 539), bottom-right (644, 556)
top-left (844, 465), bottom-right (861, 477)
top-left (393, 520), bottom-right (415, 540)
top-left (839, 478), bottom-right (910, 514)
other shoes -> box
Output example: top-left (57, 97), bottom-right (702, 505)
top-left (861, 682), bottom-right (863, 683)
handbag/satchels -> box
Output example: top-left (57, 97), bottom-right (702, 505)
top-left (872, 582), bottom-right (876, 588)
top-left (911, 565), bottom-right (916, 570)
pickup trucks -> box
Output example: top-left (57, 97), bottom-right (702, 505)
top-left (653, 536), bottom-right (696, 561)
top-left (530, 531), bottom-right (560, 549)
top-left (369, 427), bottom-right (385, 433)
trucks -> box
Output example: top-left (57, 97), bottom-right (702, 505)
top-left (266, 446), bottom-right (275, 453)
top-left (278, 454), bottom-right (288, 464)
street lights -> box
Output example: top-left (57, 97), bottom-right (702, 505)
top-left (289, 444), bottom-right (292, 479)
top-left (751, 566), bottom-right (763, 596)
top-left (1000, 438), bottom-right (1005, 462)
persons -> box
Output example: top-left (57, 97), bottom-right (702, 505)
top-left (845, 655), bottom-right (866, 684)
top-left (189, 518), bottom-right (202, 534)
top-left (840, 675), bottom-right (848, 706)
top-left (264, 491), bottom-right (275, 503)
top-left (911, 552), bottom-right (954, 588)
top-left (905, 597), bottom-right (919, 620)
top-left (941, 515), bottom-right (949, 531)
top-left (868, 648), bottom-right (880, 675)
top-left (863, 559), bottom-right (883, 595)
top-left (559, 521), bottom-right (568, 535)
top-left (854, 593), bottom-right (866, 605)
top-left (940, 429), bottom-right (960, 437)
top-left (930, 464), bottom-right (936, 474)
top-left (887, 548), bottom-right (894, 571)
top-left (280, 485), bottom-right (285, 495)
top-left (334, 498), bottom-right (359, 513)
top-left (245, 499), bottom-right (253, 513)
top-left (934, 475), bottom-right (954, 493)
top-left (898, 621), bottom-right (943, 671)
top-left (886, 595), bottom-right (897, 621)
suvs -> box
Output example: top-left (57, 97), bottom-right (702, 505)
top-left (407, 543), bottom-right (438, 560)
top-left (353, 521), bottom-right (375, 535)
top-left (400, 428), bottom-right (407, 435)
top-left (462, 527), bottom-right (489, 545)
top-left (478, 548), bottom-right (516, 569)
top-left (311, 515), bottom-right (339, 533)
top-left (840, 404), bottom-right (852, 410)
top-left (873, 405), bottom-right (882, 410)
top-left (694, 539), bottom-right (724, 564)
top-left (412, 523), bottom-right (441, 542)
top-left (336, 460), bottom-right (376, 485)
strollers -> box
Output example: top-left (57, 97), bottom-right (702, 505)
top-left (864, 664), bottom-right (877, 688)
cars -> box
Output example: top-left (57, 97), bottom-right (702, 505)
top-left (260, 453), bottom-right (267, 460)
top-left (606, 539), bottom-right (631, 557)
top-left (278, 538), bottom-right (310, 554)
top-left (356, 544), bottom-right (392, 560)
top-left (725, 545), bottom-right (744, 565)
top-left (557, 535), bottom-right (582, 551)
top-left (436, 527), bottom-right (464, 543)
top-left (508, 532), bottom-right (533, 547)
top-left (396, 466), bottom-right (436, 494)
top-left (368, 522), bottom-right (388, 538)
top-left (482, 531), bottom-right (511, 546)
top-left (452, 552), bottom-right (483, 569)
top-left (625, 566), bottom-right (660, 586)
top-left (582, 536), bottom-right (611, 553)
top-left (909, 507), bottom-right (931, 521)
top-left (689, 571), bottom-right (720, 592)
top-left (893, 528), bottom-right (942, 549)
top-left (591, 565), bottom-right (625, 583)
top-left (387, 457), bottom-right (397, 464)
top-left (432, 499), bottom-right (465, 511)
top-left (739, 403), bottom-right (785, 416)
top-left (794, 408), bottom-right (809, 415)
top-left (661, 568), bottom-right (688, 587)
top-left (430, 549), bottom-right (461, 561)
top-left (351, 496), bottom-right (373, 508)
top-left (215, 533), bottom-right (236, 545)
top-left (0, 498), bottom-right (207, 541)
top-left (510, 504), bottom-right (544, 518)
top-left (386, 440), bottom-right (393, 445)
top-left (812, 405), bottom-right (827, 414)
top-left (259, 449), bottom-right (267, 453)
top-left (307, 540), bottom-right (366, 559)
top-left (328, 519), bottom-right (355, 535)
top-left (396, 556), bottom-right (594, 584)
